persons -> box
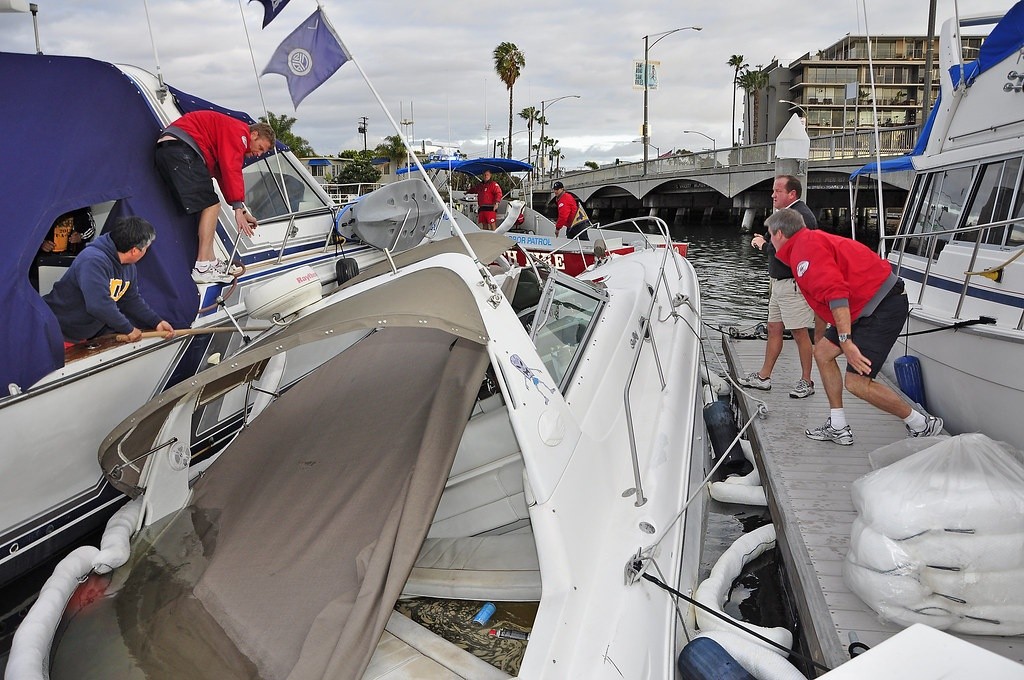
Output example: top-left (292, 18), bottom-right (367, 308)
top-left (29, 206), bottom-right (96, 294)
top-left (764, 208), bottom-right (944, 446)
top-left (463, 170), bottom-right (502, 231)
top-left (552, 182), bottom-right (591, 241)
top-left (737, 175), bottom-right (815, 398)
top-left (156, 110), bottom-right (276, 284)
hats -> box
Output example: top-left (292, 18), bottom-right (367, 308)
top-left (552, 181), bottom-right (564, 190)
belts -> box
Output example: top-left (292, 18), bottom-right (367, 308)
top-left (156, 139), bottom-right (190, 149)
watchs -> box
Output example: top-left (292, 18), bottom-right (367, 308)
top-left (838, 333), bottom-right (852, 342)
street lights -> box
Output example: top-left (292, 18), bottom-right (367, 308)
top-left (642, 25), bottom-right (704, 175)
top-left (683, 130), bottom-right (716, 169)
top-left (0, 0), bottom-right (47, 56)
top-left (541, 95), bottom-right (581, 192)
top-left (778, 99), bottom-right (808, 135)
top-left (503, 130), bottom-right (535, 159)
top-left (399, 118), bottom-right (415, 179)
top-left (632, 141), bottom-right (660, 174)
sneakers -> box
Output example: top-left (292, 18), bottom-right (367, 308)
top-left (210, 258), bottom-right (243, 274)
top-left (904, 414), bottom-right (943, 438)
top-left (804, 417), bottom-right (854, 446)
top-left (789, 378), bottom-right (815, 399)
top-left (190, 264), bottom-right (235, 284)
top-left (736, 372), bottom-right (771, 390)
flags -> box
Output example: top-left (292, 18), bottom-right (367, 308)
top-left (260, 6), bottom-right (352, 112)
top-left (248, 0), bottom-right (290, 30)
top-left (41, 217), bottom-right (174, 350)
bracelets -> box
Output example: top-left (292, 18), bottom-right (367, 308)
top-left (495, 203), bottom-right (499, 205)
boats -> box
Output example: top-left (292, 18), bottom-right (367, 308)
top-left (0, 50), bottom-right (709, 680)
top-left (847, 0), bottom-right (1023, 457)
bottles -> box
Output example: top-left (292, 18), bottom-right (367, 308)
top-left (472, 602), bottom-right (497, 626)
top-left (489, 628), bottom-right (530, 641)
